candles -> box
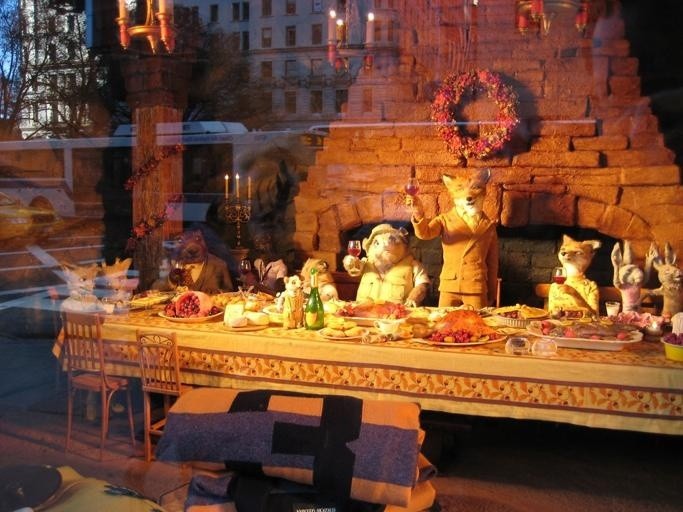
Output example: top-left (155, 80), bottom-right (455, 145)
top-left (118, 0), bottom-right (174, 26)
top-left (224, 172), bottom-right (252, 200)
top-left (328, 8), bottom-right (377, 48)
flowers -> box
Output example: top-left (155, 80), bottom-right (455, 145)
top-left (429, 67), bottom-right (520, 159)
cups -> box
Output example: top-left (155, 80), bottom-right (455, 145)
top-left (373, 319), bottom-right (398, 334)
top-left (605, 302), bottom-right (620, 317)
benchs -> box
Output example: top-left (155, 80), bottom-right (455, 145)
top-left (536, 285), bottom-right (664, 317)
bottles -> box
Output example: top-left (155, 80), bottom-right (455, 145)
top-left (304, 268), bottom-right (325, 331)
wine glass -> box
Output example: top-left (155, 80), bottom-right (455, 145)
top-left (346, 240), bottom-right (362, 274)
top-left (239, 260), bottom-right (251, 290)
top-left (552, 267), bottom-right (567, 299)
top-left (404, 177), bottom-right (419, 211)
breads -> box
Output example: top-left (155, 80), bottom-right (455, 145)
top-left (323, 319), bottom-right (366, 337)
top-left (224, 303), bottom-right (269, 327)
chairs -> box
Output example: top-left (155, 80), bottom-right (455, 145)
top-left (59, 311), bottom-right (135, 460)
top-left (133, 330), bottom-right (192, 463)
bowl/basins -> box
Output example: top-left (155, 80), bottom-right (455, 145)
top-left (659, 337), bottom-right (683, 362)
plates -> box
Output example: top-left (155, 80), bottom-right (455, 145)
top-left (157, 310), bottom-right (223, 323)
top-left (411, 306), bottom-right (644, 355)
top-left (341, 314), bottom-right (381, 327)
top-left (262, 305), bottom-right (280, 316)
top-left (215, 291), bottom-right (258, 310)
top-left (317, 325), bottom-right (371, 340)
